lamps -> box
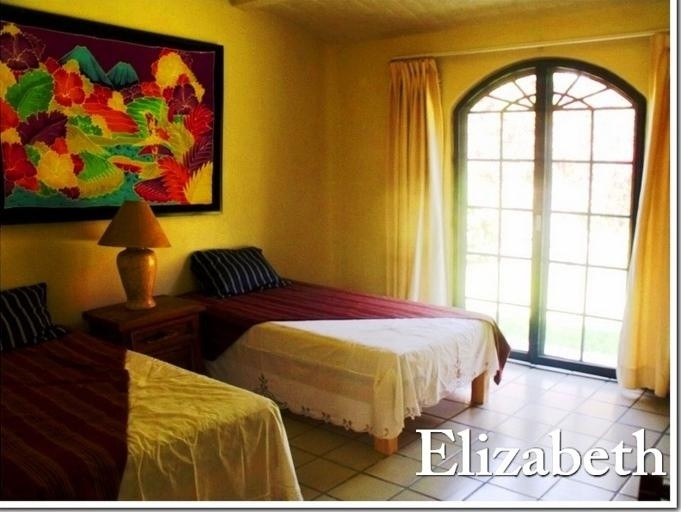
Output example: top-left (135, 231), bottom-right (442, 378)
top-left (97, 200), bottom-right (174, 311)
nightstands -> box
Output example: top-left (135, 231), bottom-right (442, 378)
top-left (82, 294), bottom-right (206, 374)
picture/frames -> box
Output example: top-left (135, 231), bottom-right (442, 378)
top-left (0, 2), bottom-right (223, 229)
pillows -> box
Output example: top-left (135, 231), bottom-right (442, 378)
top-left (0, 282), bottom-right (68, 346)
top-left (190, 247), bottom-right (294, 300)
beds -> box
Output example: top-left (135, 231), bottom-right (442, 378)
top-left (0, 329), bottom-right (306, 501)
top-left (173, 276), bottom-right (512, 454)
